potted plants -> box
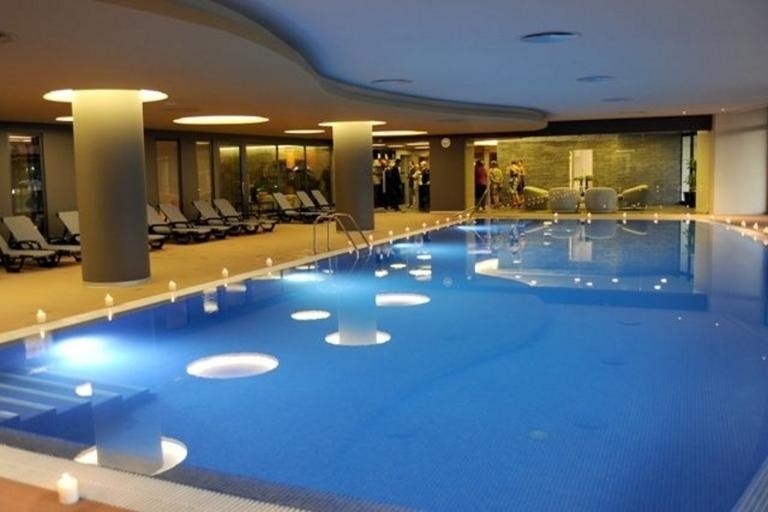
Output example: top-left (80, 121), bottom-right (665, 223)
top-left (681, 158), bottom-right (697, 208)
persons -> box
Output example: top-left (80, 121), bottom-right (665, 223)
top-left (472, 219), bottom-right (529, 258)
top-left (372, 150), bottom-right (526, 212)
top-left (248, 156), bottom-right (331, 208)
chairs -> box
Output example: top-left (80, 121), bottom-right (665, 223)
top-left (0, 188), bottom-right (335, 273)
top-left (523, 183), bottom-right (650, 213)
